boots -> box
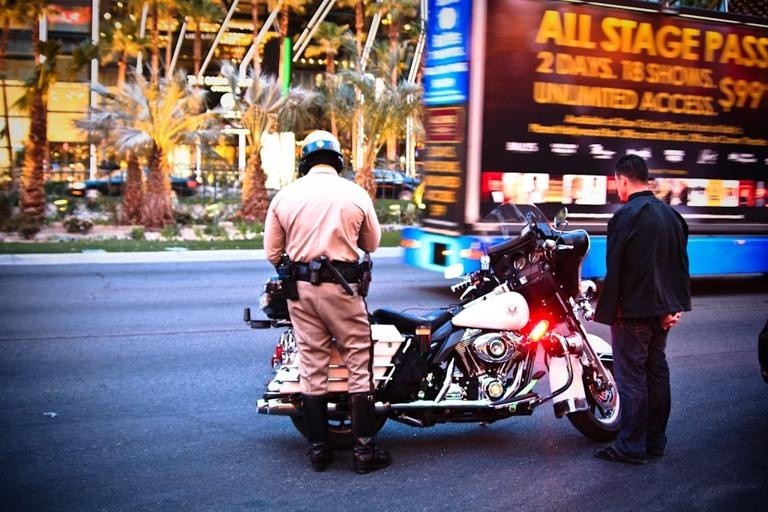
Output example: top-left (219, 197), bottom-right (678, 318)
top-left (349, 392), bottom-right (391, 474)
top-left (301, 395), bottom-right (334, 472)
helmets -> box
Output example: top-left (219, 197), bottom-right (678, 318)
top-left (299, 129), bottom-right (344, 175)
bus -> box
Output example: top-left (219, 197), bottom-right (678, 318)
top-left (399, 2), bottom-right (767, 299)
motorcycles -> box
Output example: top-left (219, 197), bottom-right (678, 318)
top-left (234, 186), bottom-right (624, 453)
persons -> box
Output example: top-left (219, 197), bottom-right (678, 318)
top-left (589, 153), bottom-right (694, 466)
top-left (262, 128), bottom-right (397, 475)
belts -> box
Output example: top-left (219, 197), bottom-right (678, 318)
top-left (295, 260), bottom-right (359, 283)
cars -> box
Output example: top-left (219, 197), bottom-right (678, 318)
top-left (63, 164), bottom-right (200, 203)
top-left (338, 148), bottom-right (426, 201)
top-left (751, 312), bottom-right (767, 387)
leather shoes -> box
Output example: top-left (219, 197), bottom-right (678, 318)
top-left (593, 446), bottom-right (643, 466)
